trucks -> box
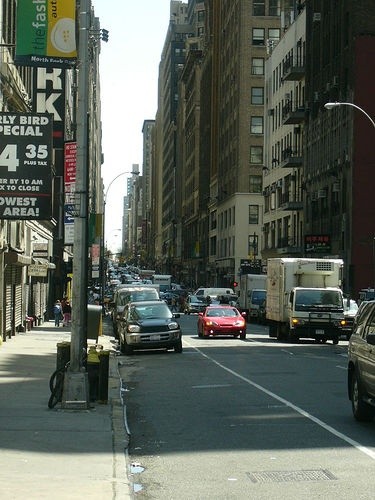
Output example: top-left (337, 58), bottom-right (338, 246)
top-left (240, 273), bottom-right (268, 324)
top-left (265, 258), bottom-right (351, 345)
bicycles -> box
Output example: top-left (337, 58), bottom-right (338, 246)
top-left (48, 347), bottom-right (87, 409)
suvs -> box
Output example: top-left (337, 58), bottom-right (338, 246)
top-left (347, 300), bottom-right (375, 423)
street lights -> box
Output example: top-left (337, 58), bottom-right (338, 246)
top-left (102, 170), bottom-right (141, 317)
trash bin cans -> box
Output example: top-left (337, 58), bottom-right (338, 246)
top-left (56, 341), bottom-right (70, 401)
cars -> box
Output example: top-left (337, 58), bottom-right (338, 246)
top-left (198, 304), bottom-right (247, 340)
top-left (343, 298), bottom-right (360, 341)
top-left (87, 260), bottom-right (240, 318)
top-left (115, 299), bottom-right (183, 355)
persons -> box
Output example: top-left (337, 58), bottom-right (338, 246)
top-left (52, 299), bottom-right (71, 328)
top-left (171, 294), bottom-right (187, 312)
